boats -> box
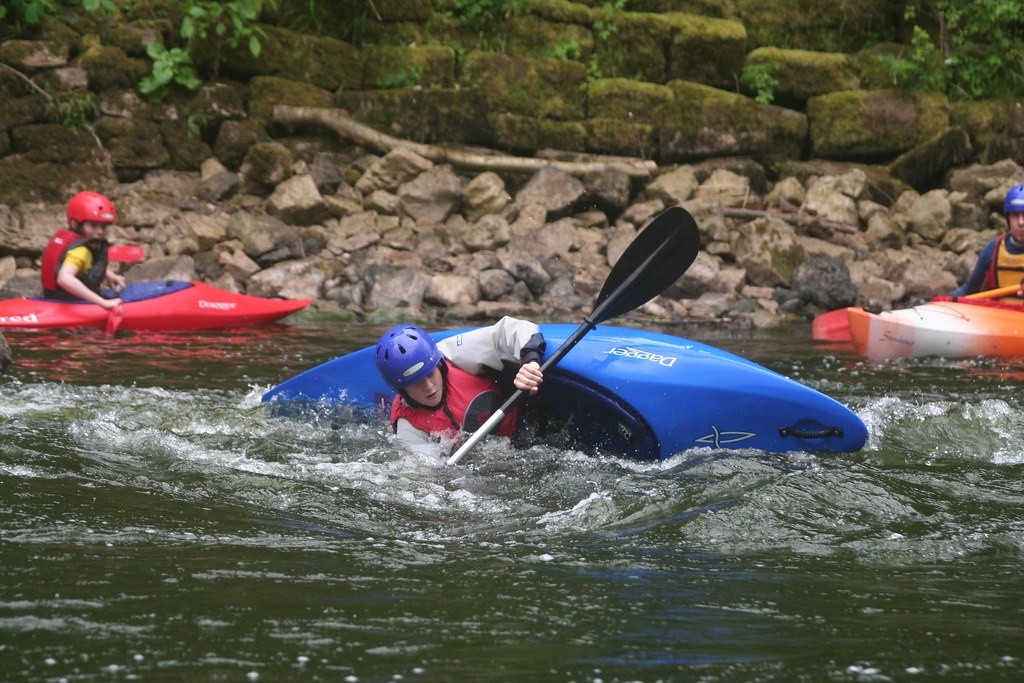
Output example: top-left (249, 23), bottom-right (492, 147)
top-left (0, 278), bottom-right (312, 341)
top-left (846, 303), bottom-right (1024, 368)
top-left (260, 321), bottom-right (870, 474)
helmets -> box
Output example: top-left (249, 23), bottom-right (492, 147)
top-left (376, 324), bottom-right (440, 387)
top-left (68, 190), bottom-right (118, 222)
top-left (1003, 183), bottom-right (1024, 214)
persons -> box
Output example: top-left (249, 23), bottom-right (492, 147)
top-left (948, 183), bottom-right (1024, 301)
top-left (40, 190), bottom-right (127, 308)
top-left (372, 317), bottom-right (547, 447)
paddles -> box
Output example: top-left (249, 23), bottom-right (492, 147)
top-left (932, 283), bottom-right (1020, 303)
top-left (446, 204), bottom-right (698, 470)
top-left (102, 243), bottom-right (145, 337)
